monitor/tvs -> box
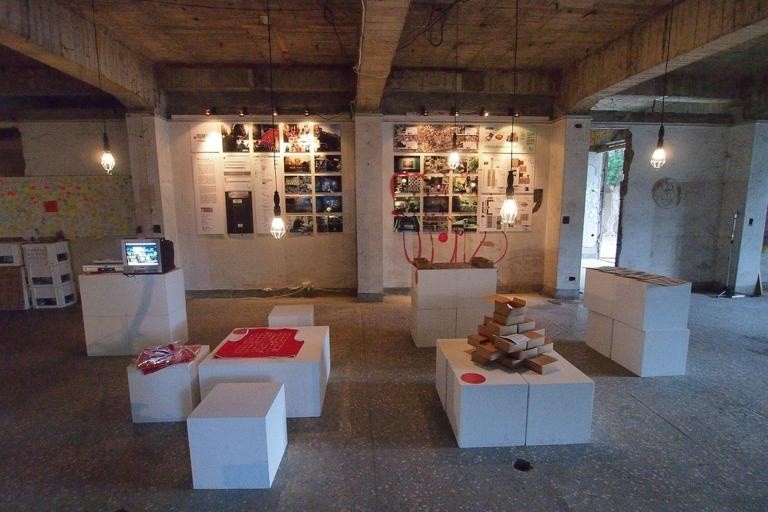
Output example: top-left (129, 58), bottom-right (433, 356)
top-left (120, 238), bottom-right (175, 274)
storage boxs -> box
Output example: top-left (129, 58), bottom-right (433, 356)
top-left (0, 236), bottom-right (77, 311)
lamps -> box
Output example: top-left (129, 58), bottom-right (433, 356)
top-left (89, 0), bottom-right (117, 176)
top-left (446, 2), bottom-right (462, 171)
top-left (649, 0), bottom-right (676, 170)
top-left (500, 2), bottom-right (519, 225)
top-left (266, 0), bottom-right (287, 241)
